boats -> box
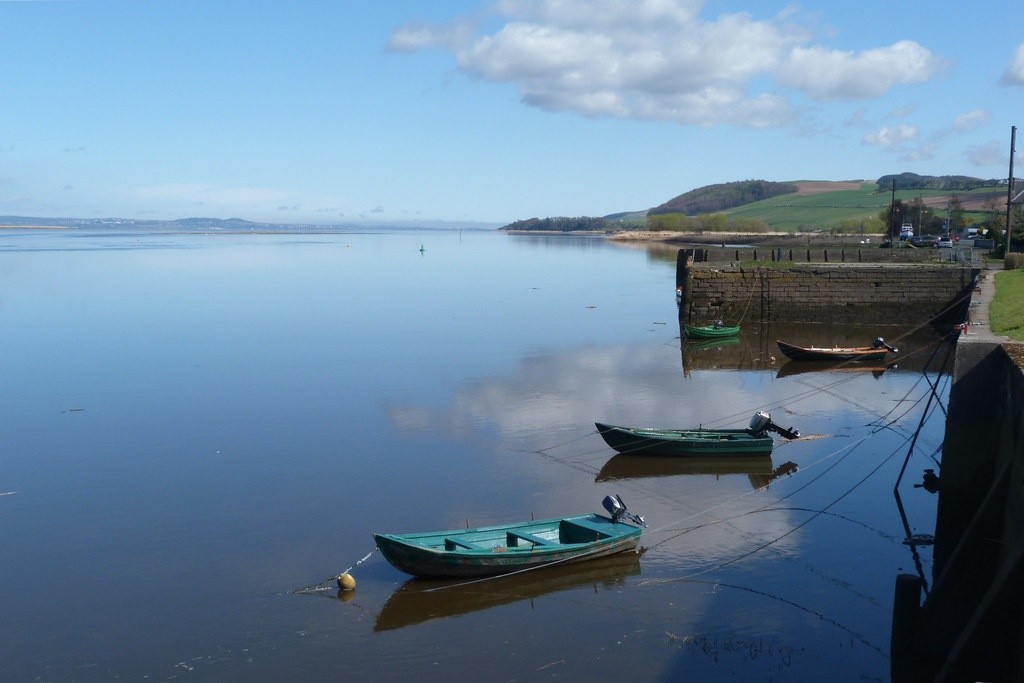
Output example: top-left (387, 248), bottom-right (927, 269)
top-left (776, 336), bottom-right (899, 361)
top-left (684, 324), bottom-right (741, 337)
top-left (371, 494), bottom-right (648, 573)
top-left (683, 337), bottom-right (741, 354)
top-left (595, 410), bottom-right (799, 456)
top-left (776, 359), bottom-right (897, 379)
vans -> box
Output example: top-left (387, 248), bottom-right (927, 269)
top-left (912, 234), bottom-right (941, 248)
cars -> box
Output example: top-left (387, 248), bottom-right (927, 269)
top-left (938, 237), bottom-right (952, 249)
